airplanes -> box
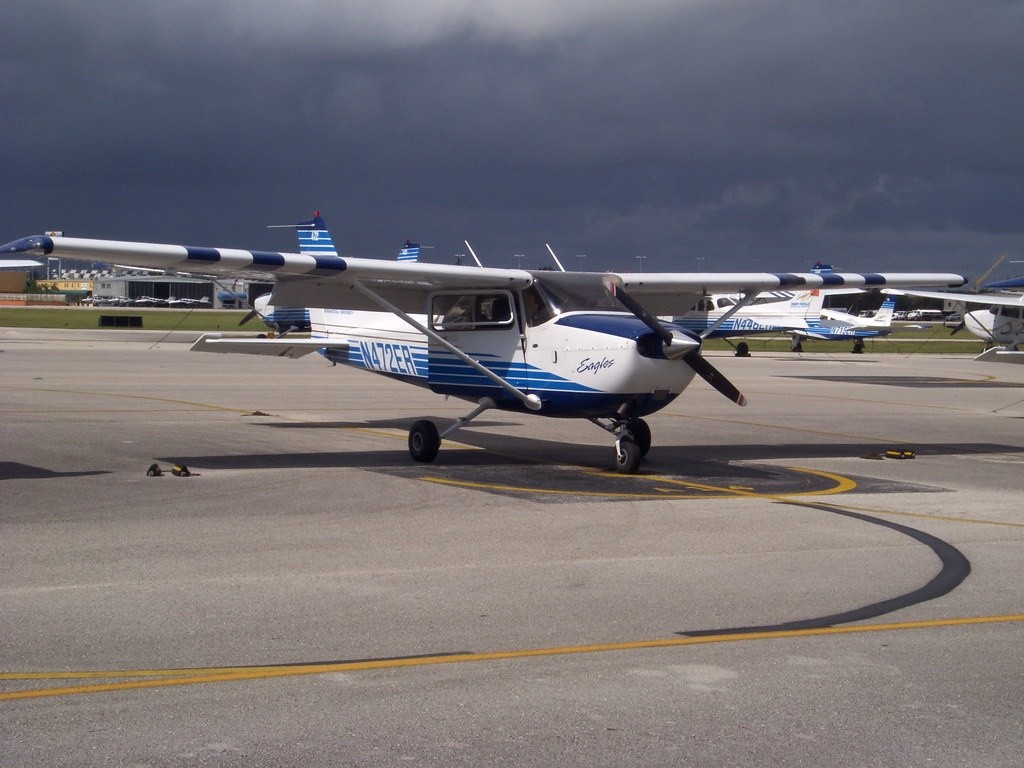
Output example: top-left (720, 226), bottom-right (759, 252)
top-left (1, 211), bottom-right (969, 475)
top-left (242, 212), bottom-right (1024, 365)
